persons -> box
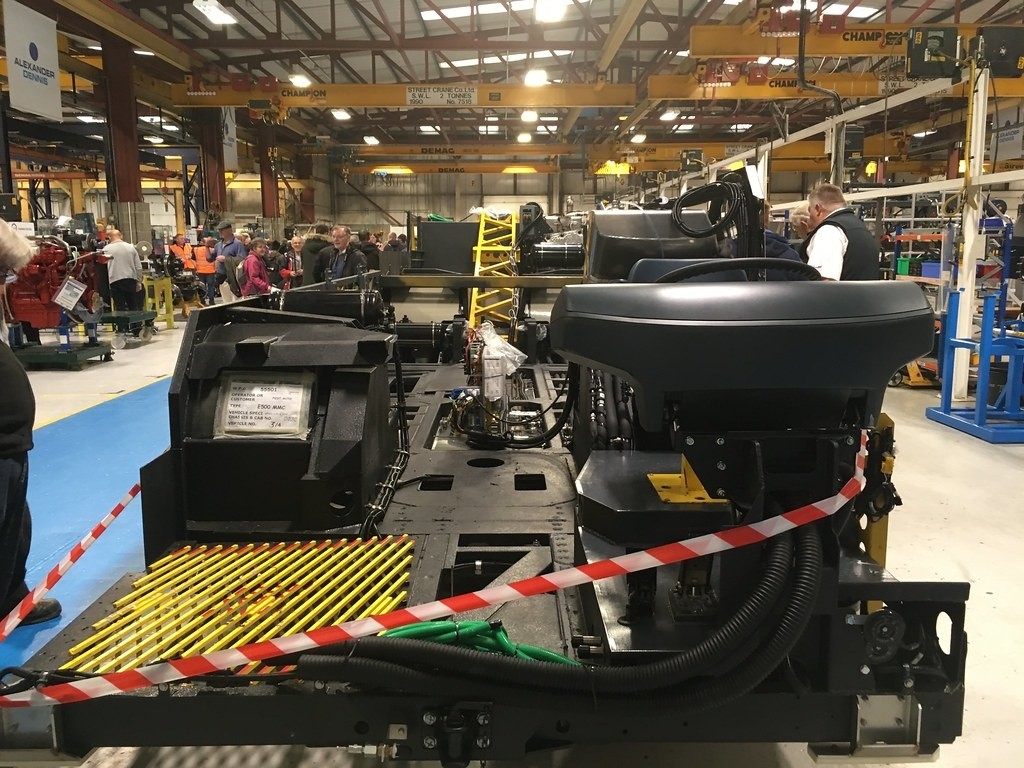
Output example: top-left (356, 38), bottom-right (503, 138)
top-left (231, 228), bottom-right (410, 306)
top-left (762, 200), bottom-right (804, 265)
top-left (804, 184), bottom-right (886, 284)
top-left (97, 229), bottom-right (149, 337)
top-left (0, 211), bottom-right (64, 632)
top-left (190, 234), bottom-right (220, 306)
top-left (10, 223), bottom-right (18, 231)
top-left (169, 233), bottom-right (210, 307)
top-left (788, 205), bottom-right (822, 251)
top-left (205, 220), bottom-right (243, 303)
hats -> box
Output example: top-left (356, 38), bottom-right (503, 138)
top-left (216, 222), bottom-right (232, 230)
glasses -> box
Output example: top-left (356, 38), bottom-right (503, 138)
top-left (0, 268), bottom-right (20, 285)
top-left (331, 233), bottom-right (349, 239)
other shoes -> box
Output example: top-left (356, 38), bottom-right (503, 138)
top-left (2, 583), bottom-right (62, 626)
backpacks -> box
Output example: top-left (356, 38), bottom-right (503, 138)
top-left (235, 254), bottom-right (257, 285)
